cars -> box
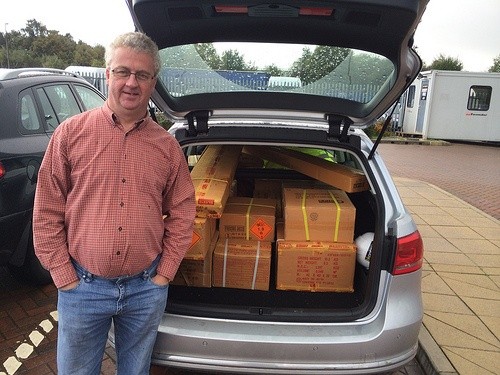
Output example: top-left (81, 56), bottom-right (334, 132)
top-left (109, 0), bottom-right (429, 372)
top-left (0, 69), bottom-right (110, 285)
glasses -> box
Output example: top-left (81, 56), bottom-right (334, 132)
top-left (110, 66), bottom-right (156, 82)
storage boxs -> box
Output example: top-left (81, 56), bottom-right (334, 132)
top-left (252, 177), bottom-right (327, 221)
top-left (282, 188), bottom-right (357, 242)
top-left (189, 145), bottom-right (242, 219)
top-left (218, 197), bottom-right (277, 243)
top-left (171, 231), bottom-right (221, 289)
top-left (242, 145), bottom-right (368, 193)
top-left (214, 242), bottom-right (272, 291)
top-left (184, 218), bottom-right (217, 260)
top-left (275, 219), bottom-right (355, 292)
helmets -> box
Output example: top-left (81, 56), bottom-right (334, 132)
top-left (355, 232), bottom-right (374, 270)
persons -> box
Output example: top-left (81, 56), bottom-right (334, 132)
top-left (32, 32), bottom-right (197, 375)
